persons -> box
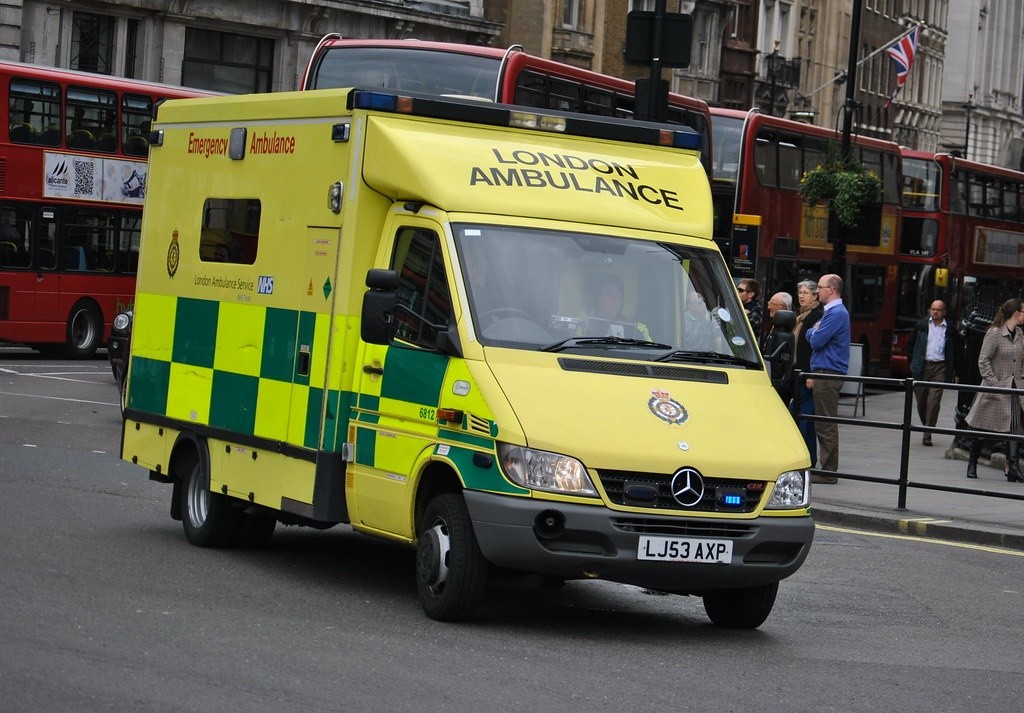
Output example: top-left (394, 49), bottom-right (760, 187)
top-left (761, 292), bottom-right (794, 408)
top-left (581, 270), bottom-right (651, 341)
top-left (966, 298), bottom-right (1024, 483)
top-left (906, 300), bottom-right (959, 447)
top-left (683, 292), bottom-right (721, 351)
top-left (806, 274), bottom-right (850, 486)
top-left (712, 278), bottom-right (763, 361)
top-left (795, 279), bottom-right (822, 469)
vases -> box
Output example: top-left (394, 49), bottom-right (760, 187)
top-left (827, 201), bottom-right (881, 247)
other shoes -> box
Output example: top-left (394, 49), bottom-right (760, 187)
top-left (922, 439), bottom-right (933, 446)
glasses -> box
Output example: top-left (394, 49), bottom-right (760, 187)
top-left (736, 288), bottom-right (750, 292)
top-left (1020, 310), bottom-right (1024, 313)
top-left (817, 286), bottom-right (836, 291)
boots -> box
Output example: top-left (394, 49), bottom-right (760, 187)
top-left (967, 438), bottom-right (983, 478)
top-left (1007, 441), bottom-right (1024, 482)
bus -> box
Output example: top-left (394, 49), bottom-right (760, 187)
top-left (1, 57), bottom-right (244, 361)
top-left (703, 103), bottom-right (907, 394)
top-left (886, 143), bottom-right (1024, 378)
top-left (294, 31), bottom-right (716, 185)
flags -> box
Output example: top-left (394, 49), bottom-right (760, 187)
top-left (884, 26), bottom-right (921, 109)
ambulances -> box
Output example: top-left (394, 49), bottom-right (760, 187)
top-left (117, 90), bottom-right (818, 634)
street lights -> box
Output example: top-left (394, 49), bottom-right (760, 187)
top-left (764, 38), bottom-right (787, 114)
top-left (962, 94), bottom-right (977, 161)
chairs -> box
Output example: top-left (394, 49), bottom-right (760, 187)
top-left (0, 242), bottom-right (87, 271)
top-left (9, 122), bottom-right (149, 157)
top-left (579, 274), bottom-right (625, 318)
top-left (120, 247), bottom-right (139, 273)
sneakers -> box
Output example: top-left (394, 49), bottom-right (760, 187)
top-left (812, 474), bottom-right (838, 484)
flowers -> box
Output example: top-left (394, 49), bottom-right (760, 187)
top-left (834, 170), bottom-right (881, 227)
top-left (798, 163), bottom-right (832, 205)
